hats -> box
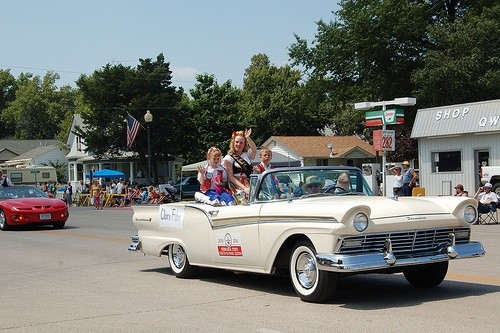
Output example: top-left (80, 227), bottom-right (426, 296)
top-left (302, 175), bottom-right (325, 193)
top-left (119, 178), bottom-right (122, 180)
top-left (483, 182), bottom-right (492, 189)
top-left (453, 184), bottom-right (464, 190)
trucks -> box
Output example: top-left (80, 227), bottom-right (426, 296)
top-left (1, 161), bottom-right (57, 190)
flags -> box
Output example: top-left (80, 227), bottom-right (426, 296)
top-left (127, 116), bottom-right (140, 147)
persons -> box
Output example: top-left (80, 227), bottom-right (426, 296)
top-left (302, 175), bottom-right (325, 194)
top-left (197, 147), bottom-right (236, 205)
top-left (0, 170), bottom-right (14, 187)
top-left (473, 183), bottom-right (498, 225)
top-left (454, 184), bottom-right (467, 196)
top-left (81, 177), bottom-right (124, 209)
top-left (401, 161), bottom-right (417, 196)
top-left (253, 149), bottom-right (286, 200)
top-left (222, 128), bottom-right (264, 204)
top-left (65, 182), bottom-right (73, 206)
top-left (389, 166), bottom-right (403, 196)
top-left (137, 168), bottom-right (142, 179)
top-left (120, 185), bottom-right (170, 207)
top-left (382, 137), bottom-right (392, 148)
top-left (43, 181), bottom-right (56, 198)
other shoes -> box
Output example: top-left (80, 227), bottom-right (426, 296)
top-left (98, 207), bottom-right (102, 210)
top-left (96, 207), bottom-right (99, 209)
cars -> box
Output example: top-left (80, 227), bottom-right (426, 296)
top-left (171, 176), bottom-right (201, 200)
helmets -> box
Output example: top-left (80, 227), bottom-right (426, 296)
top-left (401, 161), bottom-right (410, 167)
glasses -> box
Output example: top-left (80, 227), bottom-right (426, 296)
top-left (394, 168), bottom-right (399, 170)
top-left (211, 156), bottom-right (222, 161)
top-left (308, 184), bottom-right (320, 189)
top-left (455, 187), bottom-right (460, 190)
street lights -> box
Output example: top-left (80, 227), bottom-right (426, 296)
top-left (143, 110), bottom-right (154, 185)
top-left (354, 98), bottom-right (418, 196)
top-left (30, 170), bottom-right (40, 187)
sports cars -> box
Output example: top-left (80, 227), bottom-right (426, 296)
top-left (127, 152), bottom-right (485, 304)
top-left (0, 186), bottom-right (70, 230)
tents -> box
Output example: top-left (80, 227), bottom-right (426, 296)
top-left (85, 169), bottom-right (125, 184)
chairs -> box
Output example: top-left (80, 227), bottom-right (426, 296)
top-left (478, 207), bottom-right (498, 225)
